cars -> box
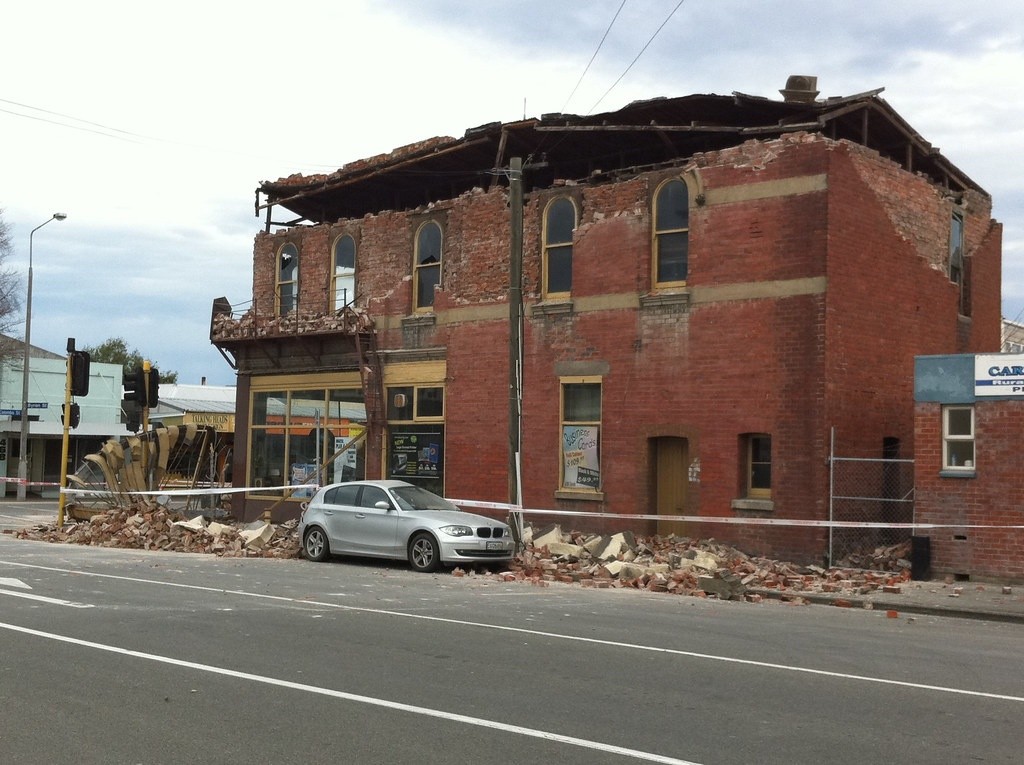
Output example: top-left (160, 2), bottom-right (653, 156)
top-left (298, 479), bottom-right (512, 571)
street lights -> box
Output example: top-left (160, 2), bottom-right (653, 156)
top-left (17, 210), bottom-right (65, 499)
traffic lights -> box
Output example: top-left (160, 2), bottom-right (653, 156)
top-left (123, 367), bottom-right (146, 406)
